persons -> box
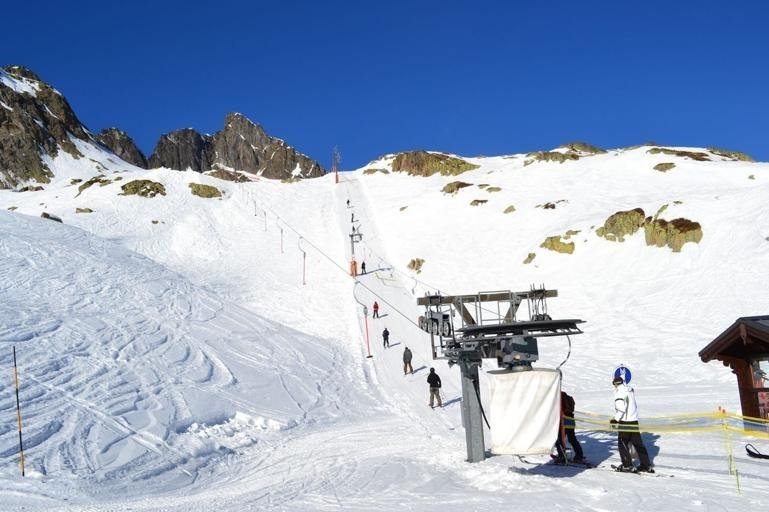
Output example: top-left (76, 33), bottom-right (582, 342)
top-left (373, 301), bottom-right (379, 319)
top-left (403, 347), bottom-right (414, 374)
top-left (428, 368), bottom-right (441, 406)
top-left (554, 392), bottom-right (583, 463)
top-left (610, 377), bottom-right (650, 470)
top-left (382, 328), bottom-right (389, 347)
top-left (362, 261), bottom-right (366, 274)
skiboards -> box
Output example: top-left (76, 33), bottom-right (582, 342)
top-left (524, 455), bottom-right (605, 468)
top-left (611, 465), bottom-right (675, 477)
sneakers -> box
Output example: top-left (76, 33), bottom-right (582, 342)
top-left (572, 456), bottom-right (587, 464)
top-left (554, 457), bottom-right (568, 465)
top-left (617, 464), bottom-right (637, 473)
top-left (639, 465), bottom-right (654, 472)
